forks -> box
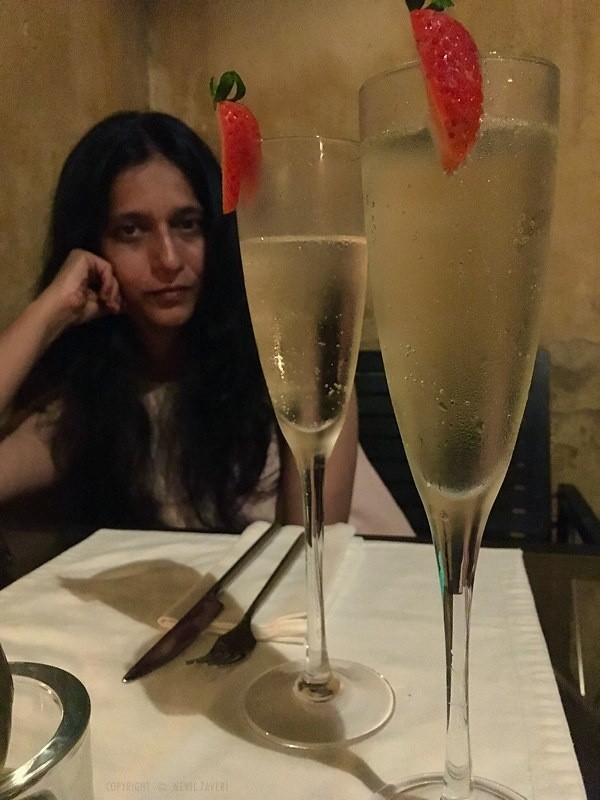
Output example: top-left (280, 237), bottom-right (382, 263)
top-left (185, 532), bottom-right (306, 669)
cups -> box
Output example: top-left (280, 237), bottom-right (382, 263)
top-left (0, 660), bottom-right (95, 800)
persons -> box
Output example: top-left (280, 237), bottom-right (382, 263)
top-left (0, 108), bottom-right (418, 537)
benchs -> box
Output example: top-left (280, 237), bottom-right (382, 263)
top-left (356, 349), bottom-right (600, 544)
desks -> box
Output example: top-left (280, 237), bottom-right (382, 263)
top-left (0, 521), bottom-right (600, 800)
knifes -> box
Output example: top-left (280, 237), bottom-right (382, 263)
top-left (121, 523), bottom-right (283, 684)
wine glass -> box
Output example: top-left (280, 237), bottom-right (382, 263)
top-left (235, 134), bottom-right (397, 753)
top-left (356, 52), bottom-right (562, 800)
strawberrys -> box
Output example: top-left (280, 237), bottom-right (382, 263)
top-left (405, 0), bottom-right (484, 174)
top-left (210, 70), bottom-right (262, 213)
